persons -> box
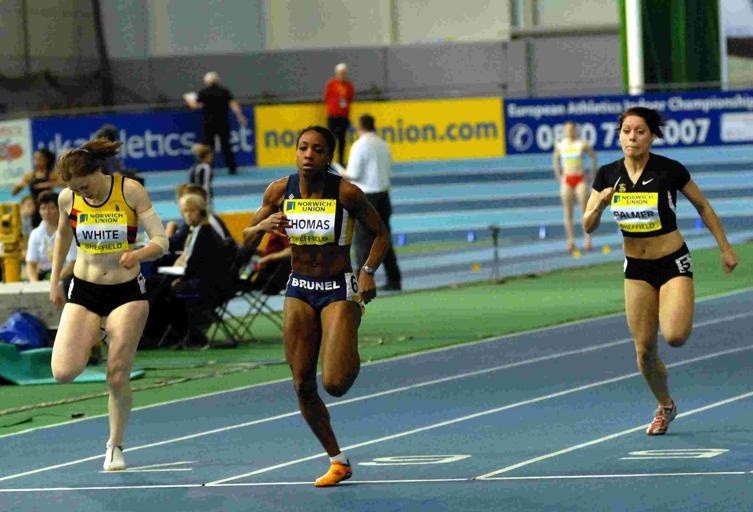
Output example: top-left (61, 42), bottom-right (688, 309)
top-left (329, 113), bottom-right (401, 291)
top-left (582, 107), bottom-right (740, 434)
top-left (14, 124), bottom-right (237, 350)
top-left (46, 137), bottom-right (168, 471)
top-left (186, 70), bottom-right (249, 173)
top-left (552, 119), bottom-right (596, 252)
top-left (242, 126), bottom-right (390, 486)
top-left (320, 62), bottom-right (356, 168)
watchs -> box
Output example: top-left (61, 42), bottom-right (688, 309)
top-left (361, 264), bottom-right (376, 278)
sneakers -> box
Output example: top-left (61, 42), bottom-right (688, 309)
top-left (102, 442), bottom-right (126, 472)
top-left (314, 458), bottom-right (354, 487)
top-left (644, 400), bottom-right (677, 435)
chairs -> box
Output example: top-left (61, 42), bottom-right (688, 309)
top-left (158, 237), bottom-right (238, 350)
top-left (225, 258), bottom-right (291, 344)
